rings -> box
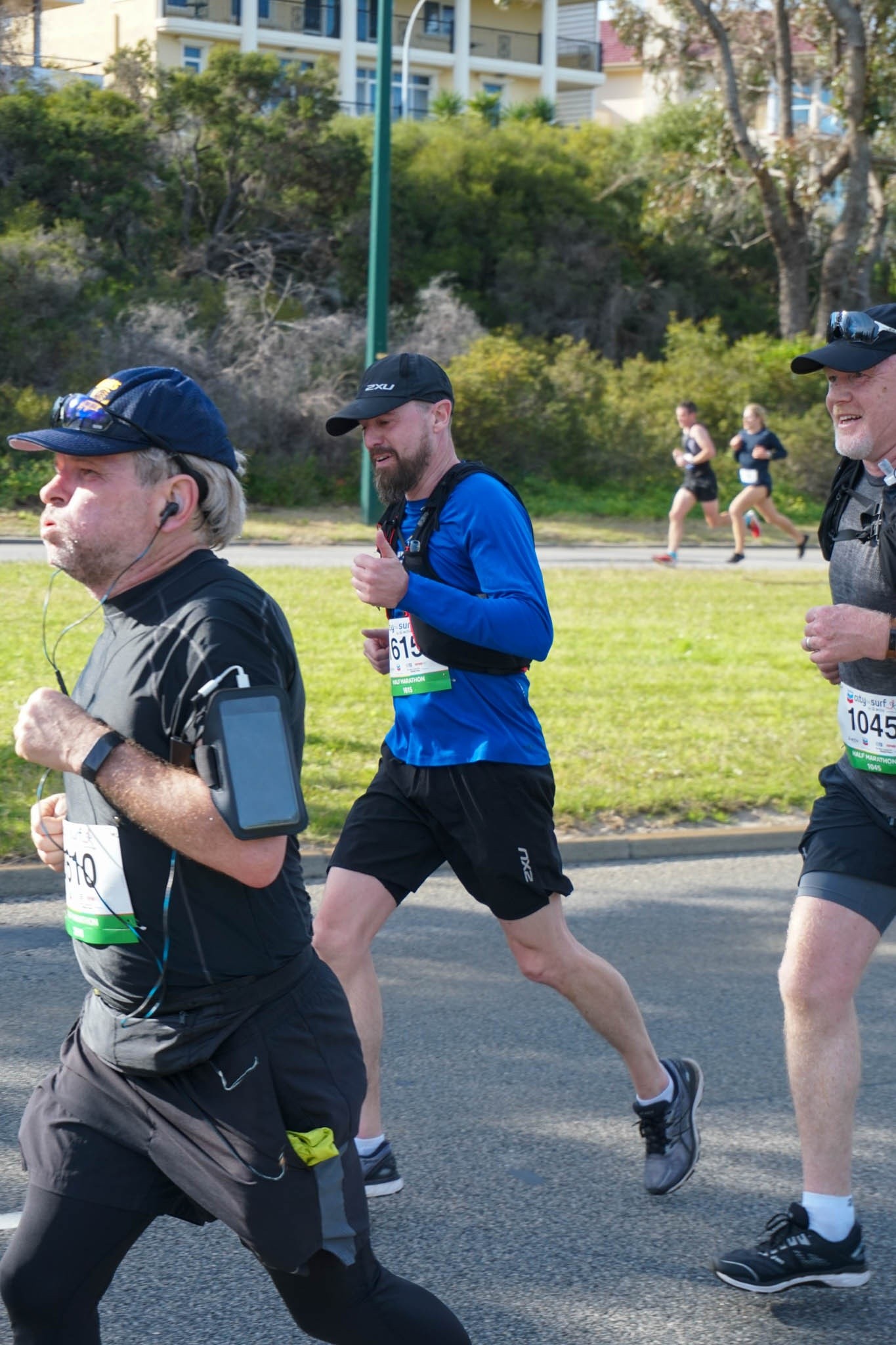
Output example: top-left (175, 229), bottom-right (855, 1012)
top-left (805, 636), bottom-right (811, 648)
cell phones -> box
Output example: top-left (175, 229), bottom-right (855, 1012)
top-left (217, 689), bottom-right (301, 830)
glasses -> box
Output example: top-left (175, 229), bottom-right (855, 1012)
top-left (51, 394), bottom-right (195, 471)
top-left (827, 311), bottom-right (896, 345)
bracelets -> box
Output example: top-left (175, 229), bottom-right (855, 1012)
top-left (81, 728), bottom-right (125, 780)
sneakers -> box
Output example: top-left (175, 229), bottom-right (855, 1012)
top-left (358, 1139), bottom-right (406, 1199)
top-left (652, 553), bottom-right (677, 569)
top-left (634, 1057), bottom-right (703, 1197)
top-left (746, 510), bottom-right (760, 539)
top-left (714, 1201), bottom-right (874, 1293)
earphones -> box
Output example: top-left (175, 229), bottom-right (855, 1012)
top-left (158, 502), bottom-right (179, 527)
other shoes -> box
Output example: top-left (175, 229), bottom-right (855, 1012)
top-left (724, 552), bottom-right (747, 566)
top-left (798, 534), bottom-right (810, 560)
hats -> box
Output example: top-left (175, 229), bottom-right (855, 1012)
top-left (789, 303), bottom-right (896, 375)
top-left (8, 363), bottom-right (246, 474)
top-left (327, 354), bottom-right (455, 437)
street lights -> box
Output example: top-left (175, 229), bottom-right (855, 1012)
top-left (401, 0), bottom-right (508, 124)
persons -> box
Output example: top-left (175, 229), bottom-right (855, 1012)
top-left (310, 350), bottom-right (705, 1196)
top-left (651, 401), bottom-right (762, 569)
top-left (711, 300), bottom-right (896, 1295)
top-left (724, 403), bottom-right (810, 565)
top-left (0, 358), bottom-right (471, 1345)
